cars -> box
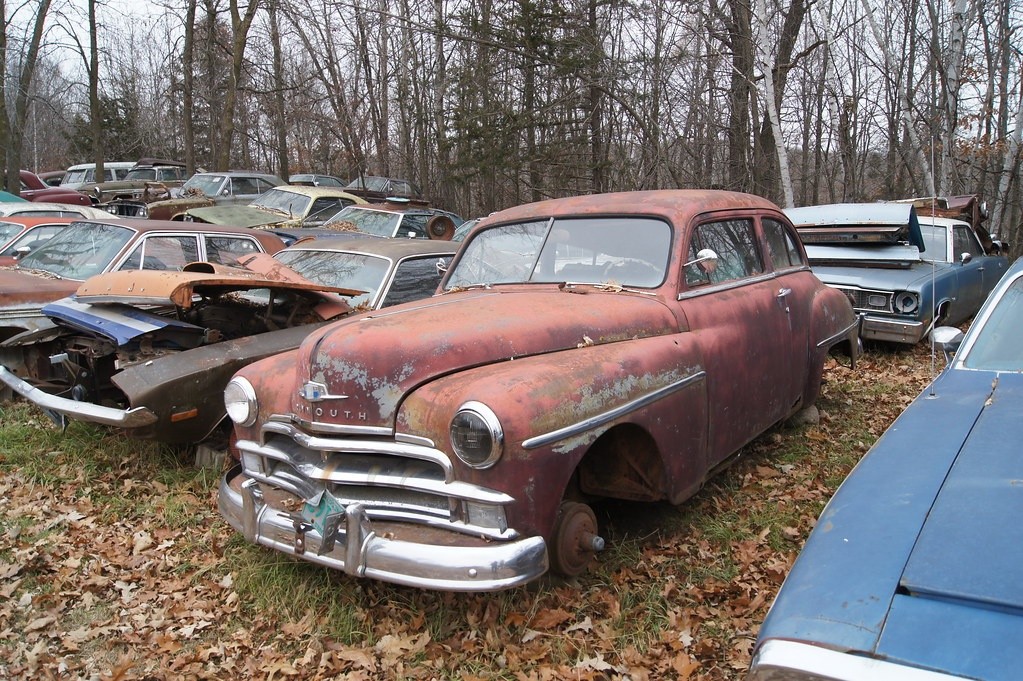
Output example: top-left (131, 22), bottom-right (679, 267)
top-left (777, 192), bottom-right (1012, 343)
top-left (218, 188), bottom-right (863, 594)
top-left (0, 158), bottom-right (500, 448)
top-left (749, 257), bottom-right (1023, 681)
